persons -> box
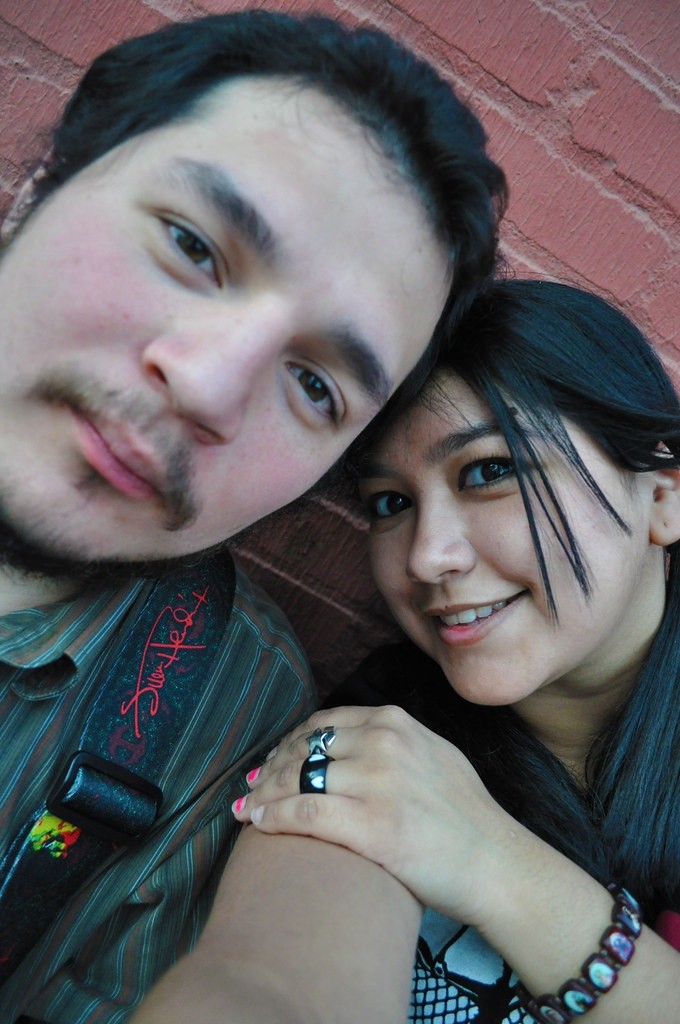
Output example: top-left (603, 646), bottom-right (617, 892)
top-left (123, 275), bottom-right (679, 1024)
top-left (0, 8), bottom-right (509, 1024)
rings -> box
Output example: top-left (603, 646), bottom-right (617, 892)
top-left (299, 753), bottom-right (336, 794)
top-left (306, 723), bottom-right (336, 756)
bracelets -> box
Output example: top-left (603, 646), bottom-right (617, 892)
top-left (512, 882), bottom-right (643, 1024)
